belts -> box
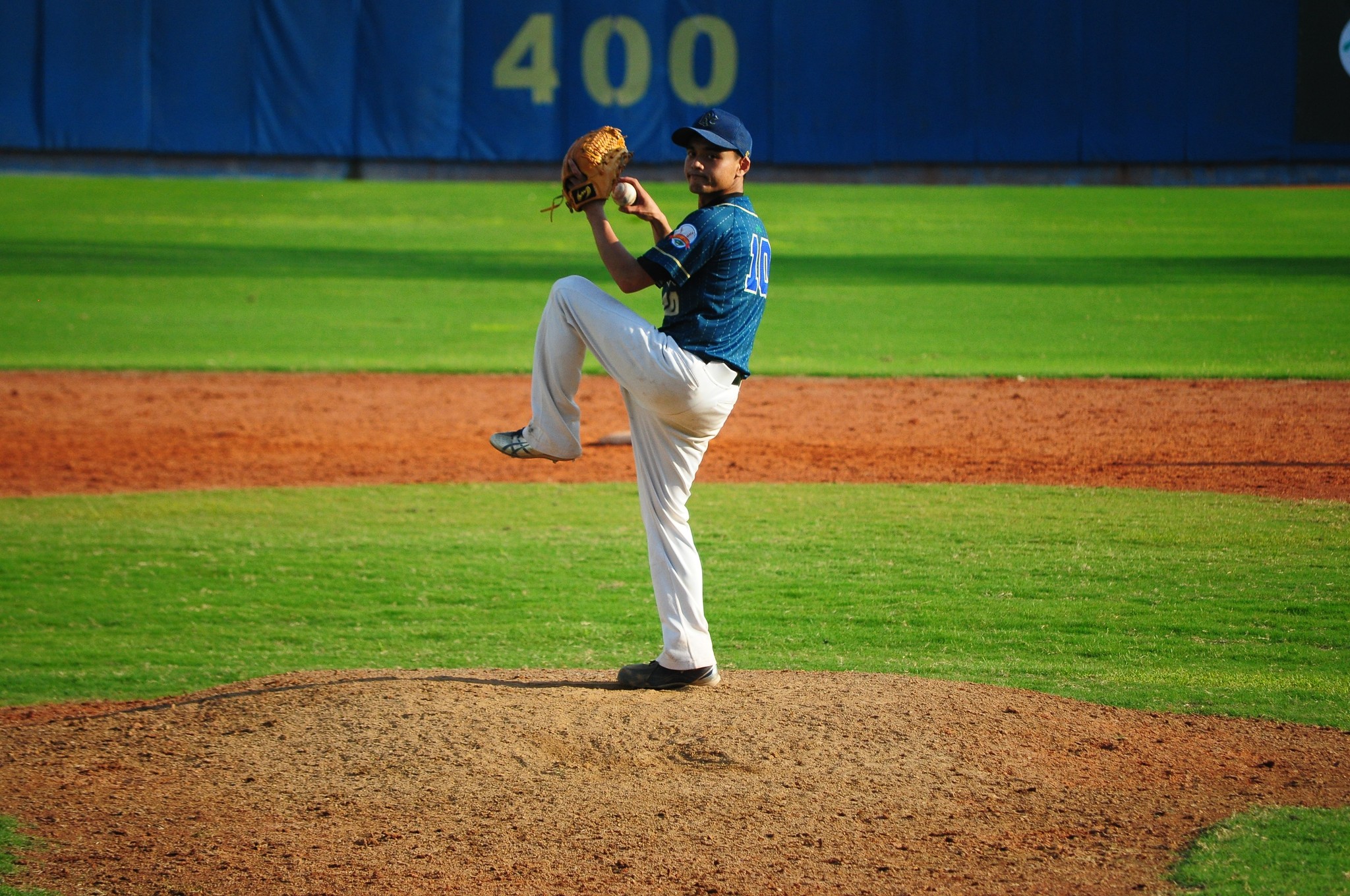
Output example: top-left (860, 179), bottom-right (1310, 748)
top-left (697, 354), bottom-right (743, 386)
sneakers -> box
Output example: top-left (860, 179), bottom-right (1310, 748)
top-left (488, 427), bottom-right (577, 465)
top-left (617, 660), bottom-right (721, 691)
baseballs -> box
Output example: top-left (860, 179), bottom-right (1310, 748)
top-left (611, 182), bottom-right (636, 206)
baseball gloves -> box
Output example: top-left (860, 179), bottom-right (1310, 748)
top-left (562, 126), bottom-right (629, 212)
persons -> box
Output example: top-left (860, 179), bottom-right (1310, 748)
top-left (489, 106), bottom-right (772, 690)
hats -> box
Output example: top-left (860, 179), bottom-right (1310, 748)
top-left (671, 107), bottom-right (752, 158)
